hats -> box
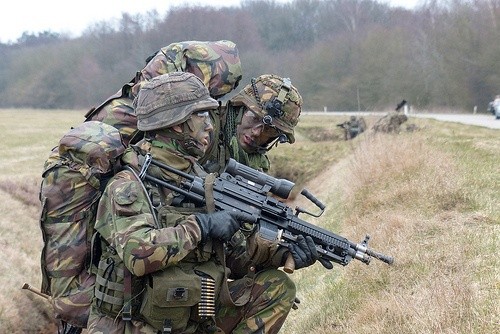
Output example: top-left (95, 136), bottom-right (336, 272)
top-left (228, 74), bottom-right (303, 144)
top-left (132, 72), bottom-right (220, 132)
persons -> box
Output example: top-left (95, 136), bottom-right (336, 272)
top-left (87, 71), bottom-right (333, 334)
top-left (343, 115), bottom-right (367, 141)
top-left (196, 74), bottom-right (302, 310)
top-left (373, 113), bottom-right (408, 134)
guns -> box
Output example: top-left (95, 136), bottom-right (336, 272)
top-left (138, 153), bottom-right (394, 274)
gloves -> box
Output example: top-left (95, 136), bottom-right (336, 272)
top-left (288, 234), bottom-right (318, 269)
top-left (195, 209), bottom-right (256, 244)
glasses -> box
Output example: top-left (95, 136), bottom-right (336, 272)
top-left (188, 111), bottom-right (212, 125)
top-left (242, 108), bottom-right (281, 138)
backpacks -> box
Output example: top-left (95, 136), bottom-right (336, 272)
top-left (83, 37), bottom-right (243, 149)
top-left (38, 121), bottom-right (132, 329)
